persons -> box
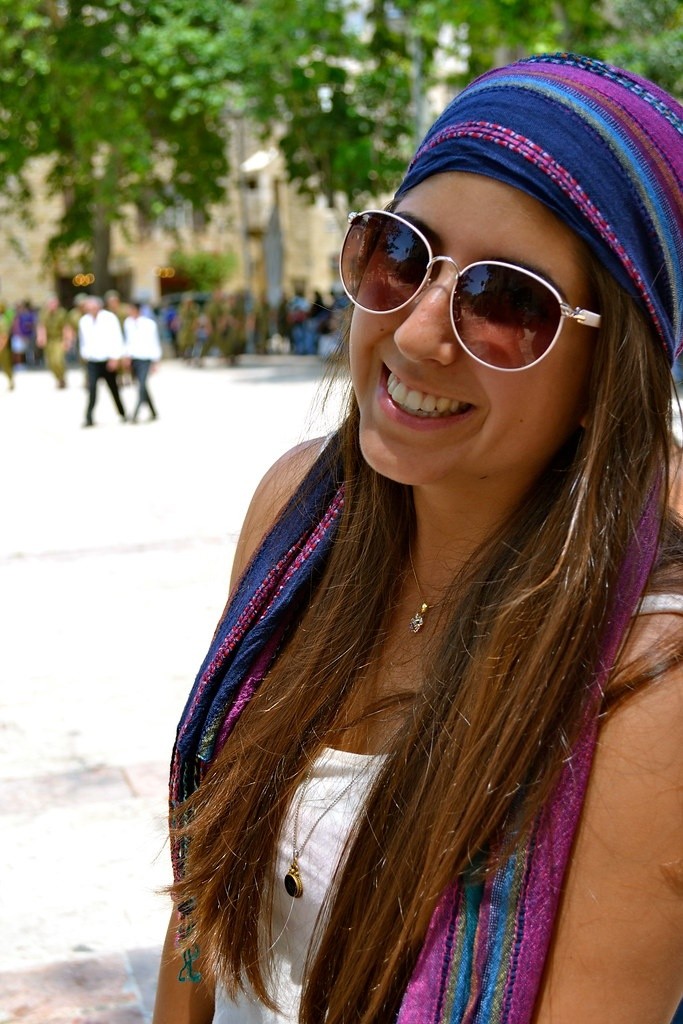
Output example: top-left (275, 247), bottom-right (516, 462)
top-left (153, 286), bottom-right (350, 369)
top-left (0, 290), bottom-right (132, 391)
top-left (152, 52), bottom-right (683, 1024)
top-left (123, 303), bottom-right (162, 422)
top-left (77, 295), bottom-right (126, 427)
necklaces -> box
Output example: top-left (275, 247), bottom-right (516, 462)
top-left (284, 725), bottom-right (405, 899)
top-left (407, 539), bottom-right (457, 633)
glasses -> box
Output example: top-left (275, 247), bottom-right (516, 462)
top-left (339, 210), bottom-right (602, 372)
